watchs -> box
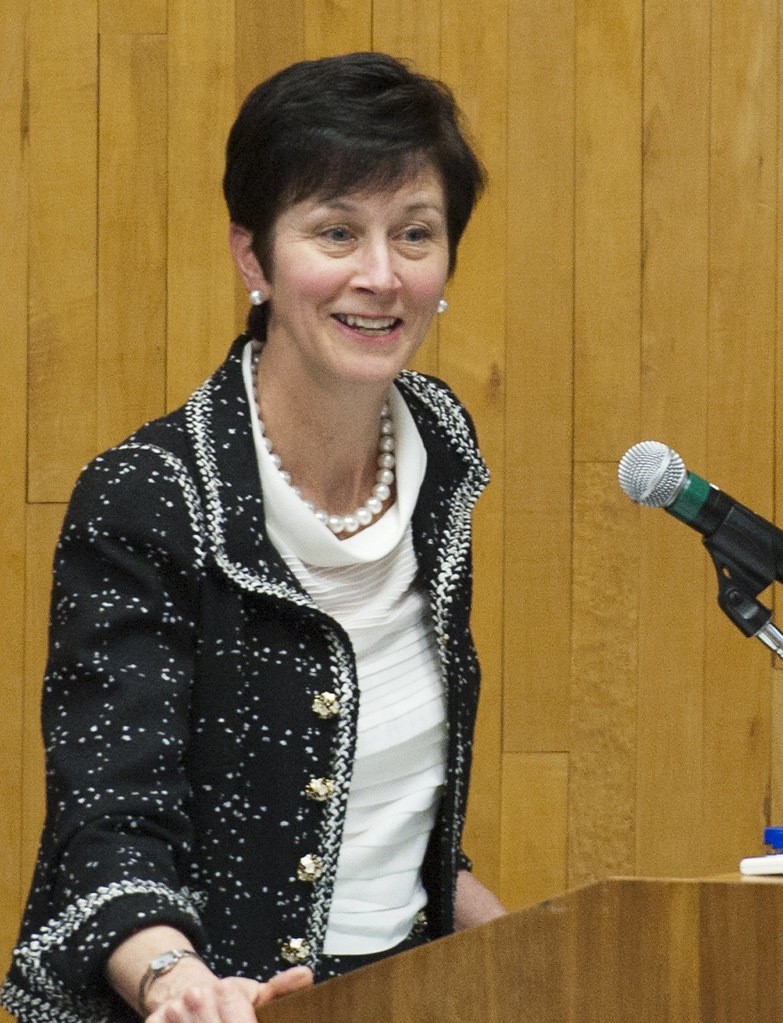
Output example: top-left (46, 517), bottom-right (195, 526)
top-left (138, 948), bottom-right (204, 1014)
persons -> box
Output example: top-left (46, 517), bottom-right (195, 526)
top-left (0, 51), bottom-right (508, 1023)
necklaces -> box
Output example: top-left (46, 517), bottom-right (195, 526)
top-left (249, 352), bottom-right (395, 535)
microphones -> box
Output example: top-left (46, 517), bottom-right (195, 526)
top-left (618, 439), bottom-right (783, 586)
